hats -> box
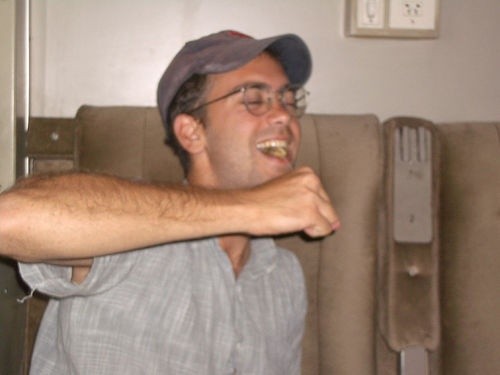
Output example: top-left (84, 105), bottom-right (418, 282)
top-left (157, 30), bottom-right (312, 132)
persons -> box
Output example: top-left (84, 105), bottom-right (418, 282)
top-left (0, 30), bottom-right (342, 375)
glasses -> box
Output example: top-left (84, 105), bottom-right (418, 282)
top-left (186, 84), bottom-right (310, 119)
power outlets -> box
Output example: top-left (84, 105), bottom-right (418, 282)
top-left (345, 0), bottom-right (442, 37)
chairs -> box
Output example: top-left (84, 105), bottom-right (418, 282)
top-left (74, 107), bottom-right (500, 375)
